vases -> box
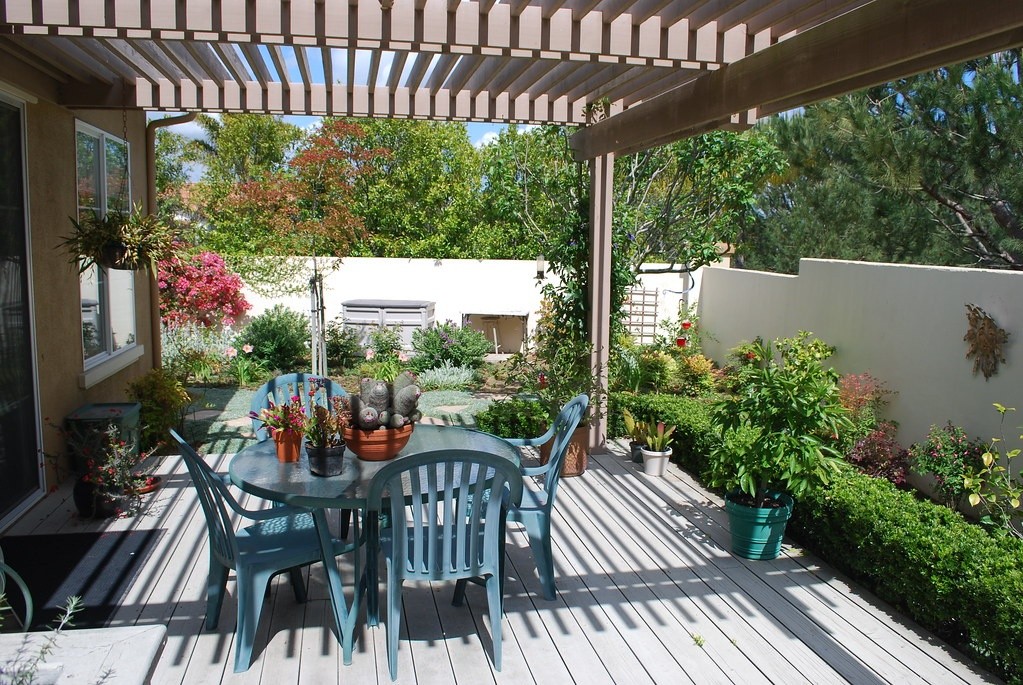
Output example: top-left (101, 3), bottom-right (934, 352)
top-left (273, 431), bottom-right (301, 462)
top-left (304, 443), bottom-right (345, 476)
top-left (72, 476), bottom-right (128, 517)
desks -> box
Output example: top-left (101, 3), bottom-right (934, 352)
top-left (230, 425), bottom-right (521, 530)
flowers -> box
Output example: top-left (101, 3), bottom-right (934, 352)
top-left (158, 241), bottom-right (264, 383)
top-left (33, 407), bottom-right (168, 516)
top-left (304, 401), bottom-right (347, 447)
top-left (414, 317), bottom-right (491, 368)
top-left (836, 371), bottom-right (988, 503)
top-left (249, 396), bottom-right (309, 431)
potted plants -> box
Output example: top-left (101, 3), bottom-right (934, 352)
top-left (703, 342), bottom-right (859, 560)
top-left (510, 339), bottom-right (619, 478)
top-left (641, 421), bottom-right (677, 476)
top-left (52, 206), bottom-right (170, 278)
top-left (336, 370), bottom-right (423, 461)
top-left (624, 407), bottom-right (650, 463)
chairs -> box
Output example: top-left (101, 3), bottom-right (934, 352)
top-left (165, 426), bottom-right (359, 673)
top-left (466, 394), bottom-right (590, 602)
top-left (342, 448), bottom-right (526, 681)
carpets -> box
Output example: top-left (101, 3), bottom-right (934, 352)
top-left (0, 528), bottom-right (167, 634)
